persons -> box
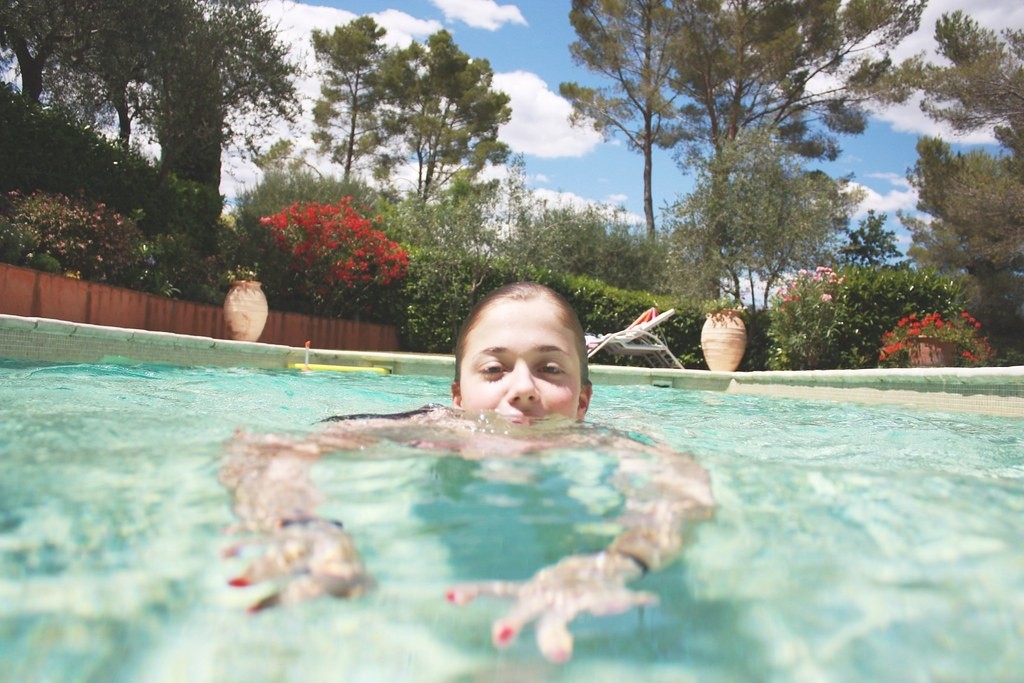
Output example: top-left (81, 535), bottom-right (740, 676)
top-left (448, 282), bottom-right (593, 423)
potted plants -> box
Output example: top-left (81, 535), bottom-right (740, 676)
top-left (701, 297), bottom-right (749, 372)
top-left (222, 263), bottom-right (269, 342)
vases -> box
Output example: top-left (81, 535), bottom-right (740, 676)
top-left (908, 333), bottom-right (957, 367)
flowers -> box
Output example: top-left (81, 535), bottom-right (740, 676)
top-left (880, 310), bottom-right (991, 363)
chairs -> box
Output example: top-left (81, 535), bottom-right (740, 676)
top-left (586, 307), bottom-right (688, 369)
